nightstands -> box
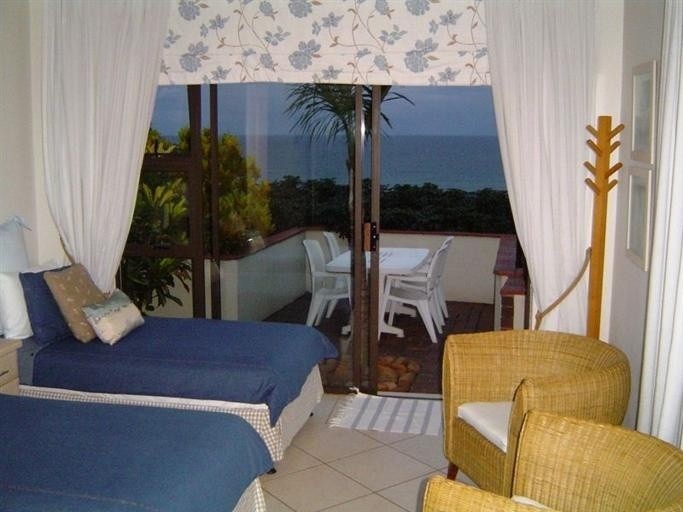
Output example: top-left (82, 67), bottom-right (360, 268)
top-left (0, 337), bottom-right (22, 398)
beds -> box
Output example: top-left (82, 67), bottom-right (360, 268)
top-left (1, 391), bottom-right (276, 511)
top-left (0, 216), bottom-right (338, 463)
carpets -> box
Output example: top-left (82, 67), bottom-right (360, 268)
top-left (327, 387), bottom-right (439, 437)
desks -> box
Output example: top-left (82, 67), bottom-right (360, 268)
top-left (493, 234), bottom-right (523, 332)
top-left (326, 246), bottom-right (429, 340)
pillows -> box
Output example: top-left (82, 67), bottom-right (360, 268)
top-left (83, 288), bottom-right (148, 346)
top-left (0, 272), bottom-right (33, 342)
top-left (41, 263), bottom-right (106, 344)
top-left (17, 267), bottom-right (71, 346)
top-left (0, 217), bottom-right (28, 272)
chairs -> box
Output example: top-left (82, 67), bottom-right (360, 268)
top-left (439, 330), bottom-right (632, 497)
top-left (501, 277), bottom-right (527, 331)
top-left (322, 231), bottom-right (349, 321)
top-left (387, 234), bottom-right (453, 327)
top-left (378, 244), bottom-right (451, 343)
top-left (300, 238), bottom-right (350, 329)
top-left (418, 408), bottom-right (682, 512)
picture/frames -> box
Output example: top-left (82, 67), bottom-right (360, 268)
top-left (621, 167), bottom-right (650, 273)
top-left (630, 60), bottom-right (656, 164)
